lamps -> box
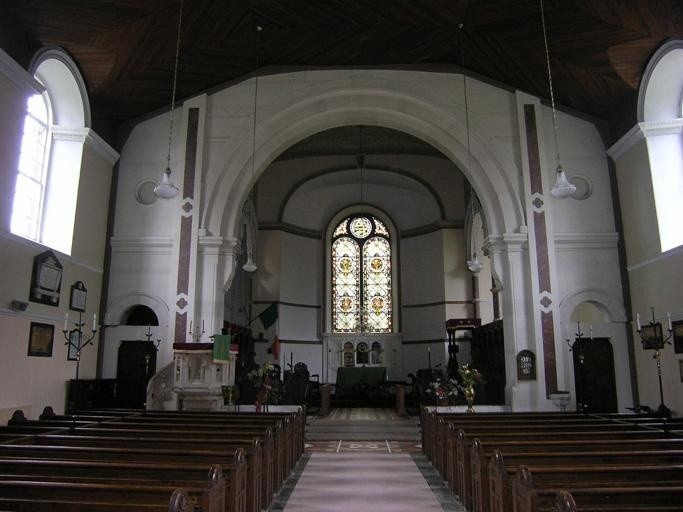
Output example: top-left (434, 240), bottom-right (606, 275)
top-left (242, 26), bottom-right (262, 272)
top-left (458, 24), bottom-right (484, 272)
top-left (540, 0), bottom-right (577, 198)
top-left (153, 0), bottom-right (183, 199)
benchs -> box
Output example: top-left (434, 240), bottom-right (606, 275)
top-left (1, 411), bottom-right (303, 512)
top-left (422, 408), bottom-right (683, 512)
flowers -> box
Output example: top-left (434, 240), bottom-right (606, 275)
top-left (456, 364), bottom-right (484, 383)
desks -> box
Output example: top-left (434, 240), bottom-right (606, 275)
top-left (335, 367), bottom-right (386, 399)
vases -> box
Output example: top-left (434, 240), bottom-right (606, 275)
top-left (464, 381), bottom-right (476, 413)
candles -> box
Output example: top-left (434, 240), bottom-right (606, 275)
top-left (637, 314), bottom-right (641, 330)
top-left (668, 312), bottom-right (672, 329)
top-left (203, 319), bottom-right (204, 330)
top-left (190, 320), bottom-right (192, 332)
top-left (65, 320), bottom-right (67, 330)
top-left (591, 325), bottom-right (592, 339)
top-left (94, 319), bottom-right (95, 330)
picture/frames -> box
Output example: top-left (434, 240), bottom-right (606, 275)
top-left (671, 320), bottom-right (683, 353)
top-left (641, 323), bottom-right (664, 349)
top-left (67, 328), bottom-right (83, 361)
top-left (28, 322), bottom-right (55, 357)
